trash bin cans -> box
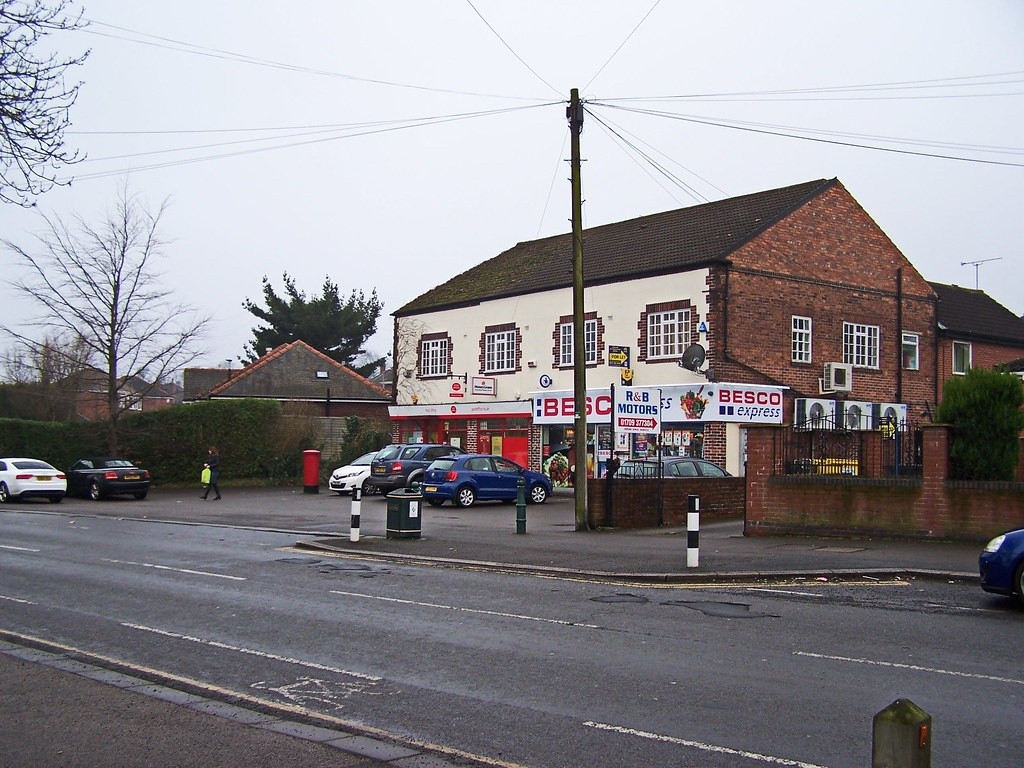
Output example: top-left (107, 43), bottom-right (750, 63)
top-left (303, 449), bottom-right (320, 496)
top-left (385, 488), bottom-right (424, 540)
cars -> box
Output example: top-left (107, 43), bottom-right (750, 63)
top-left (328, 452), bottom-right (377, 498)
top-left (0, 458), bottom-right (68, 505)
top-left (68, 459), bottom-right (150, 501)
top-left (978, 529), bottom-right (1024, 595)
top-left (613, 457), bottom-right (735, 478)
top-left (423, 454), bottom-right (553, 509)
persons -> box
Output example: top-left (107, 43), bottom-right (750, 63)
top-left (198, 446), bottom-right (222, 500)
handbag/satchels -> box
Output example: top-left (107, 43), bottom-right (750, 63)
top-left (201, 468), bottom-right (211, 483)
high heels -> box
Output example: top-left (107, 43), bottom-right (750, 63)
top-left (213, 494), bottom-right (221, 500)
top-left (200, 494), bottom-right (207, 500)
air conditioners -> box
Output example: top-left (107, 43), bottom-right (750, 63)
top-left (872, 403), bottom-right (907, 432)
top-left (824, 362), bottom-right (852, 393)
top-left (836, 400), bottom-right (872, 430)
top-left (793, 398), bottom-right (836, 429)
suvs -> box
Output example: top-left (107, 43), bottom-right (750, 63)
top-left (371, 443), bottom-right (469, 498)
